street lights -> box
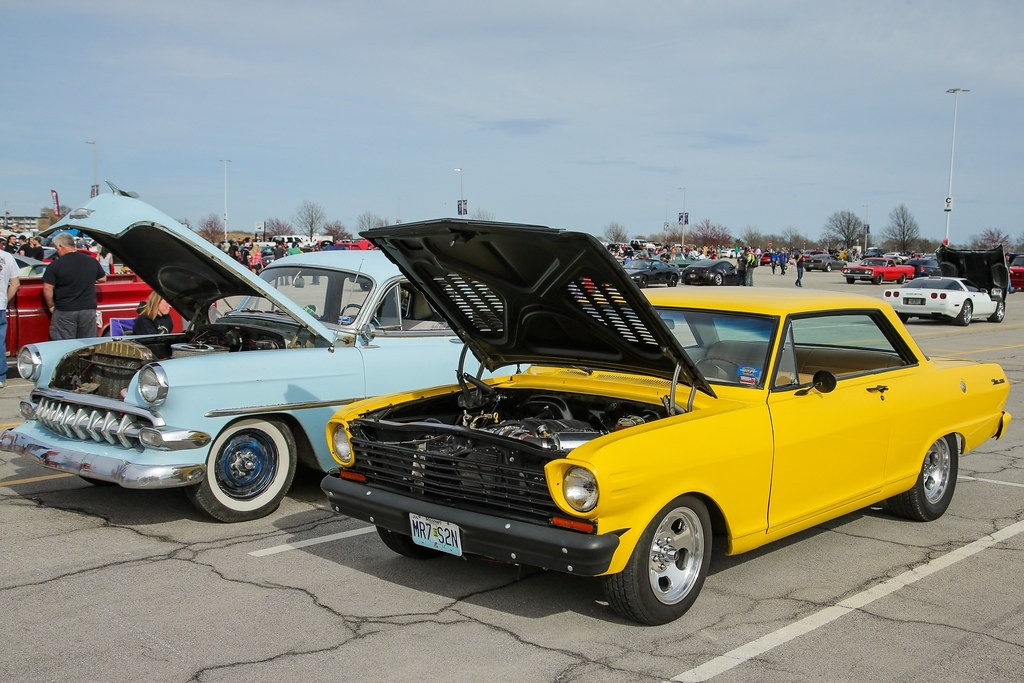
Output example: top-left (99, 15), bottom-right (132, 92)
top-left (219, 160), bottom-right (231, 241)
top-left (84, 141), bottom-right (97, 196)
top-left (454, 168), bottom-right (464, 219)
top-left (945, 89), bottom-right (971, 246)
top-left (678, 187), bottom-right (686, 246)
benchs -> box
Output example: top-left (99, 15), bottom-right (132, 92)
top-left (703, 341), bottom-right (903, 387)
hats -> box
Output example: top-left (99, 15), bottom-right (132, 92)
top-left (16, 235), bottom-right (26, 240)
top-left (663, 249), bottom-right (667, 253)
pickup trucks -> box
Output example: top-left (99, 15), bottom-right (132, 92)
top-left (5, 264), bottom-right (193, 357)
top-left (245, 235), bottom-right (310, 252)
top-left (841, 257), bottom-right (915, 285)
top-left (721, 249), bottom-right (741, 258)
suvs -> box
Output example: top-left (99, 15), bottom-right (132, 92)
top-left (629, 239), bottom-right (648, 246)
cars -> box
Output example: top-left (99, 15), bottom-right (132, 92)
top-left (760, 253), bottom-right (771, 265)
top-left (682, 259), bottom-right (739, 286)
top-left (320, 217), bottom-right (1012, 627)
top-left (0, 179), bottom-right (534, 524)
top-left (883, 242), bottom-right (1010, 326)
top-left (621, 258), bottom-right (679, 288)
top-left (903, 258), bottom-right (941, 278)
top-left (864, 249), bottom-right (935, 265)
top-left (803, 254), bottom-right (843, 272)
top-left (1008, 255), bottom-right (1024, 290)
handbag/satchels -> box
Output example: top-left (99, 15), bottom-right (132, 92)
top-left (747, 253), bottom-right (758, 267)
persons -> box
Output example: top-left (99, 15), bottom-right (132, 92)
top-left (133, 291), bottom-right (173, 334)
top-left (607, 240), bottom-right (915, 287)
top-left (216, 237), bottom-right (302, 274)
top-left (310, 246), bottom-right (316, 252)
top-left (41, 232), bottom-right (113, 341)
top-left (1005, 252), bottom-right (1016, 293)
top-left (0, 232), bottom-right (44, 261)
top-left (0, 249), bottom-right (20, 387)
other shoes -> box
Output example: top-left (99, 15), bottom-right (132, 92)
top-left (0, 380), bottom-right (8, 388)
top-left (795, 282), bottom-right (802, 287)
top-left (781, 272), bottom-right (785, 275)
